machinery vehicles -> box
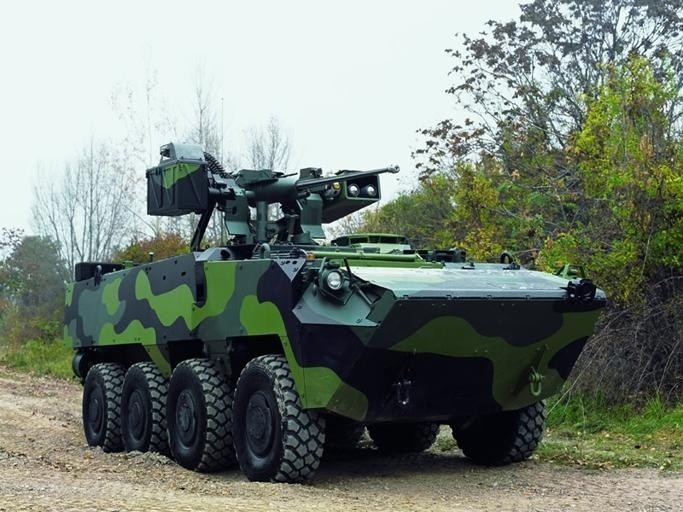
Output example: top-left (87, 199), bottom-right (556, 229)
top-left (60, 141), bottom-right (608, 480)
